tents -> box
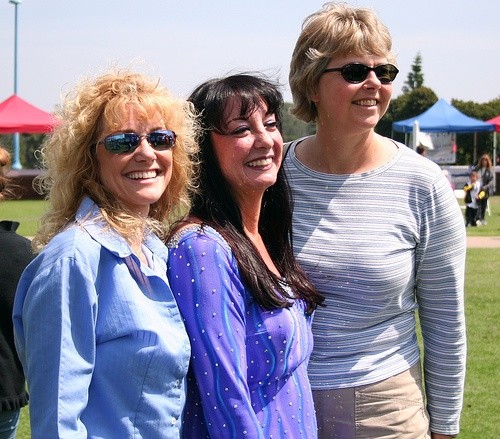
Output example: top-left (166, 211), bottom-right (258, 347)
top-left (0, 94), bottom-right (62, 133)
top-left (391, 97), bottom-right (500, 193)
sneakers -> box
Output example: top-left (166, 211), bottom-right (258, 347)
top-left (476, 218), bottom-right (487, 226)
top-left (488, 212), bottom-right (491, 216)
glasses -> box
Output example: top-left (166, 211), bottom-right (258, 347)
top-left (321, 61), bottom-right (399, 85)
top-left (91, 129), bottom-right (178, 154)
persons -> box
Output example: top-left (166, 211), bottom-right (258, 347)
top-left (0, 148), bottom-right (42, 439)
top-left (416, 146), bottom-right (426, 156)
top-left (463, 154), bottom-right (495, 227)
top-left (283, 2), bottom-right (467, 439)
top-left (165, 74), bottom-right (328, 439)
top-left (12, 69), bottom-right (211, 439)
